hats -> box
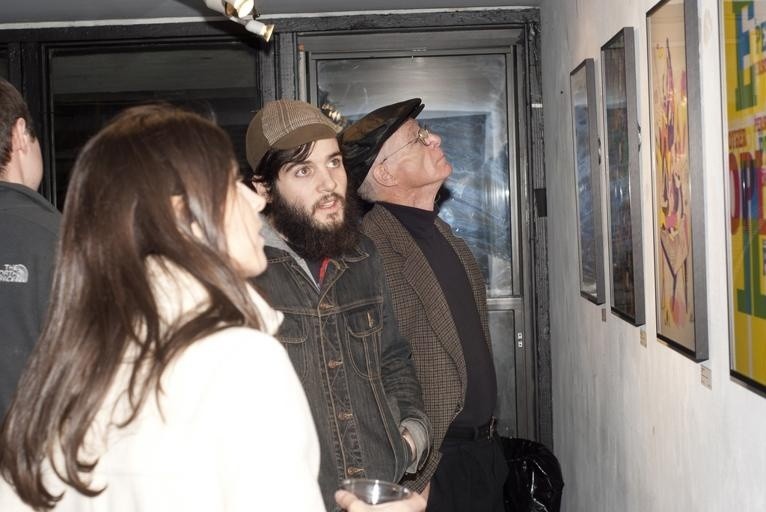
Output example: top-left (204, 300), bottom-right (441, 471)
top-left (335, 97), bottom-right (424, 194)
top-left (245, 99), bottom-right (343, 170)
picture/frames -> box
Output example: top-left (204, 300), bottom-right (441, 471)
top-left (566, 58), bottom-right (607, 307)
top-left (645, 1), bottom-right (709, 365)
top-left (717, 1), bottom-right (765, 402)
top-left (598, 26), bottom-right (645, 327)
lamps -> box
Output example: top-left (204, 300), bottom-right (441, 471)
top-left (203, 0), bottom-right (275, 44)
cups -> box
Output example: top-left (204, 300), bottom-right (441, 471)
top-left (338, 478), bottom-right (412, 506)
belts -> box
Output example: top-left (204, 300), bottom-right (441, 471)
top-left (450, 420), bottom-right (496, 443)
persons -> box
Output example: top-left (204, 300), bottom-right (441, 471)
top-left (336, 97), bottom-right (505, 511)
top-left (0, 108), bottom-right (429, 512)
top-left (1, 75), bottom-right (63, 420)
top-left (247, 101), bottom-right (431, 511)
